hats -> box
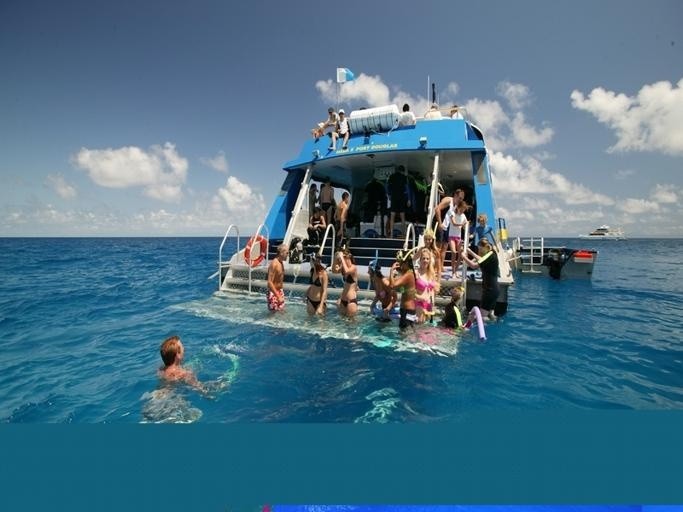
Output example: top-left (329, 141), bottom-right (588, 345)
top-left (431, 103), bottom-right (439, 109)
top-left (338, 108), bottom-right (345, 114)
top-left (328, 107), bottom-right (334, 113)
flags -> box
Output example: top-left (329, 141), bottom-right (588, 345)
top-left (336, 67), bottom-right (354, 83)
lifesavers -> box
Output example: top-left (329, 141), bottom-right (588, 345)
top-left (245, 235), bottom-right (267, 268)
top-left (374, 304), bottom-right (401, 319)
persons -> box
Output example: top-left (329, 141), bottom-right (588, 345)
top-left (307, 176), bottom-right (334, 244)
top-left (423, 104), bottom-right (441, 119)
top-left (327, 109), bottom-right (349, 149)
top-left (424, 171), bottom-right (444, 211)
top-left (444, 288), bottom-right (474, 328)
top-left (367, 260), bottom-right (397, 311)
top-left (303, 253), bottom-right (328, 315)
top-left (413, 229), bottom-right (441, 282)
top-left (449, 202), bottom-right (467, 278)
top-left (332, 250), bottom-right (358, 315)
top-left (266, 244), bottom-right (288, 311)
top-left (470, 215), bottom-right (497, 275)
top-left (334, 192), bottom-right (352, 250)
top-left (430, 191), bottom-right (465, 271)
top-left (136, 335), bottom-right (218, 422)
top-left (448, 105), bottom-right (462, 119)
top-left (398, 104), bottom-right (416, 125)
top-left (390, 248), bottom-right (436, 322)
top-left (320, 107), bottom-right (338, 128)
top-left (461, 239), bottom-right (498, 315)
top-left (364, 165), bottom-right (411, 235)
